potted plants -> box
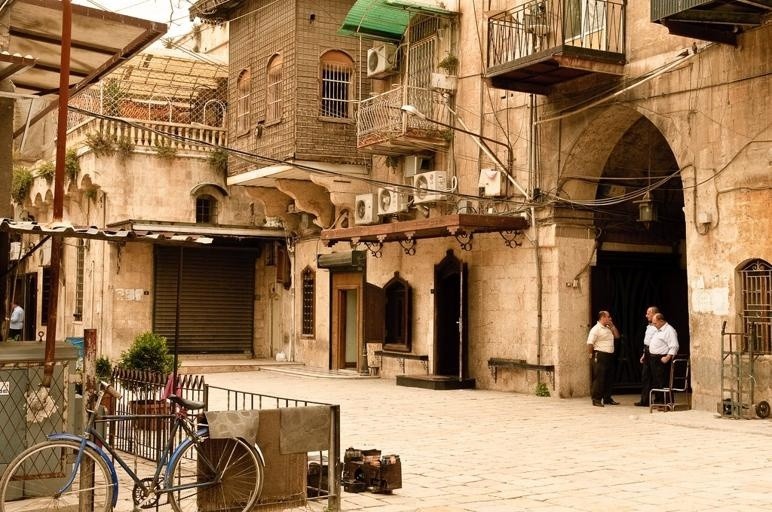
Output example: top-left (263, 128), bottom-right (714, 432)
top-left (94, 332), bottom-right (179, 432)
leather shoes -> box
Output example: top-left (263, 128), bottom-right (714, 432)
top-left (610, 401), bottom-right (619, 405)
top-left (634, 402), bottom-right (648, 406)
top-left (653, 399), bottom-right (664, 403)
top-left (594, 403), bottom-right (604, 407)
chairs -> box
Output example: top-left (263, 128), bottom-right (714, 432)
top-left (649, 354), bottom-right (690, 413)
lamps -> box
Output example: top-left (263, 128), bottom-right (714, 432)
top-left (636, 191), bottom-right (660, 228)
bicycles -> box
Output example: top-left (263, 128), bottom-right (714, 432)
top-left (0, 373), bottom-right (265, 511)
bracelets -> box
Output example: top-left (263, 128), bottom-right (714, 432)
top-left (665, 356), bottom-right (670, 361)
top-left (611, 326), bottom-right (616, 330)
top-left (588, 353), bottom-right (593, 358)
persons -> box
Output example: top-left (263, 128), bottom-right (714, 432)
top-left (587, 309), bottom-right (624, 407)
top-left (649, 314), bottom-right (680, 411)
top-left (631, 306), bottom-right (660, 406)
top-left (4, 300), bottom-right (32, 341)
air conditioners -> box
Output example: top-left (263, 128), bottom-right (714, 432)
top-left (355, 170), bottom-right (447, 226)
top-left (367, 43), bottom-right (397, 80)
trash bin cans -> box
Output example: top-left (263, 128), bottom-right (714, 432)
top-left (67, 337), bottom-right (84, 357)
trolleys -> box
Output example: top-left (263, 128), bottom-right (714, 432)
top-left (713, 319), bottom-right (770, 421)
